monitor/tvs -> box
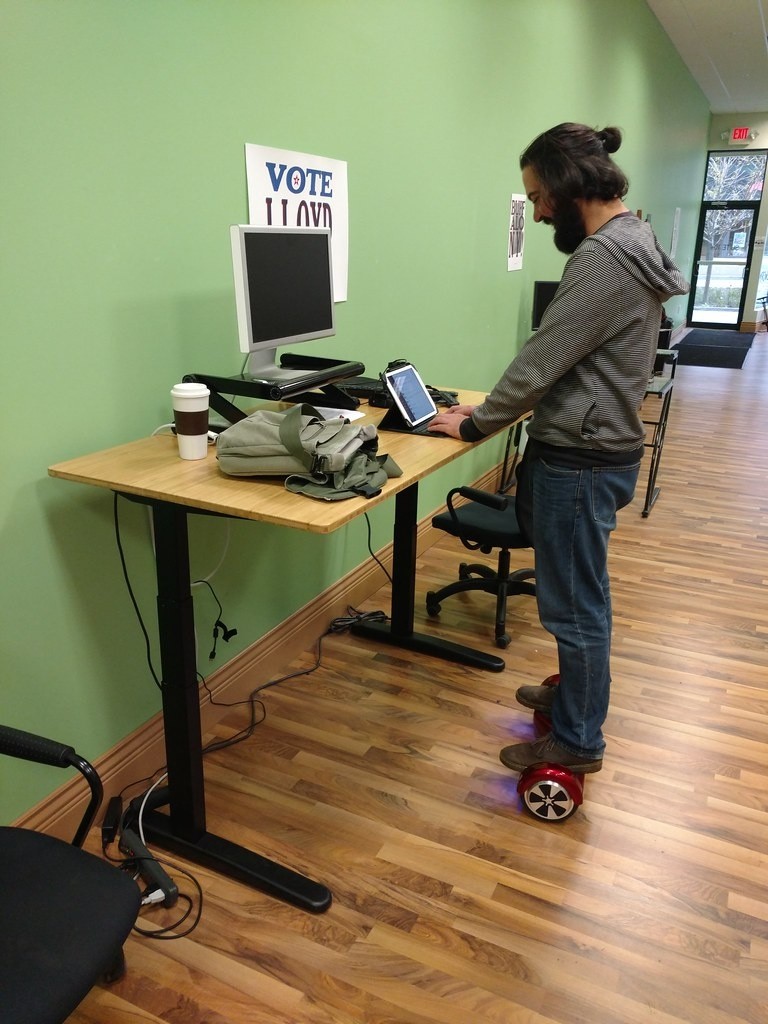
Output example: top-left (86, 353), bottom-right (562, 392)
top-left (229, 223), bottom-right (337, 382)
top-left (531, 281), bottom-right (562, 331)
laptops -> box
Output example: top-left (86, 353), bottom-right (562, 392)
top-left (383, 364), bottom-right (455, 439)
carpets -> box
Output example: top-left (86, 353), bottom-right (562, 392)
top-left (663, 329), bottom-right (757, 369)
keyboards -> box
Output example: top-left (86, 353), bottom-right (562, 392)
top-left (340, 384), bottom-right (459, 400)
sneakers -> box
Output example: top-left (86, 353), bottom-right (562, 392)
top-left (515, 686), bottom-right (560, 712)
top-left (499, 732), bottom-right (602, 773)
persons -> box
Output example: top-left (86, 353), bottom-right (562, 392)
top-left (425, 121), bottom-right (690, 775)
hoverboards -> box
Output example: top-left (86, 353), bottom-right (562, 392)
top-left (517, 672), bottom-right (585, 824)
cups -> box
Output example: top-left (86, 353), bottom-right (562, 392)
top-left (170, 383), bottom-right (210, 460)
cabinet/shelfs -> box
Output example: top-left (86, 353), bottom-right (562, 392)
top-left (495, 349), bottom-right (679, 518)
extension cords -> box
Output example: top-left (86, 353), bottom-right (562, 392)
top-left (118, 830), bottom-right (178, 909)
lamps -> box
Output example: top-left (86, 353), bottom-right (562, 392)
top-left (720, 128), bottom-right (759, 145)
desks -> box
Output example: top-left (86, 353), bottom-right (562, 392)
top-left (48, 385), bottom-right (533, 911)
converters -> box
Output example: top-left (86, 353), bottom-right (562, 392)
top-left (102, 796), bottom-right (123, 843)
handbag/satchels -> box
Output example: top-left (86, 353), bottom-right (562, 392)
top-left (216, 403), bottom-right (404, 500)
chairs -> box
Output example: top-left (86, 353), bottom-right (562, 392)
top-left (425, 486), bottom-right (538, 649)
top-left (0, 724), bottom-right (142, 1024)
